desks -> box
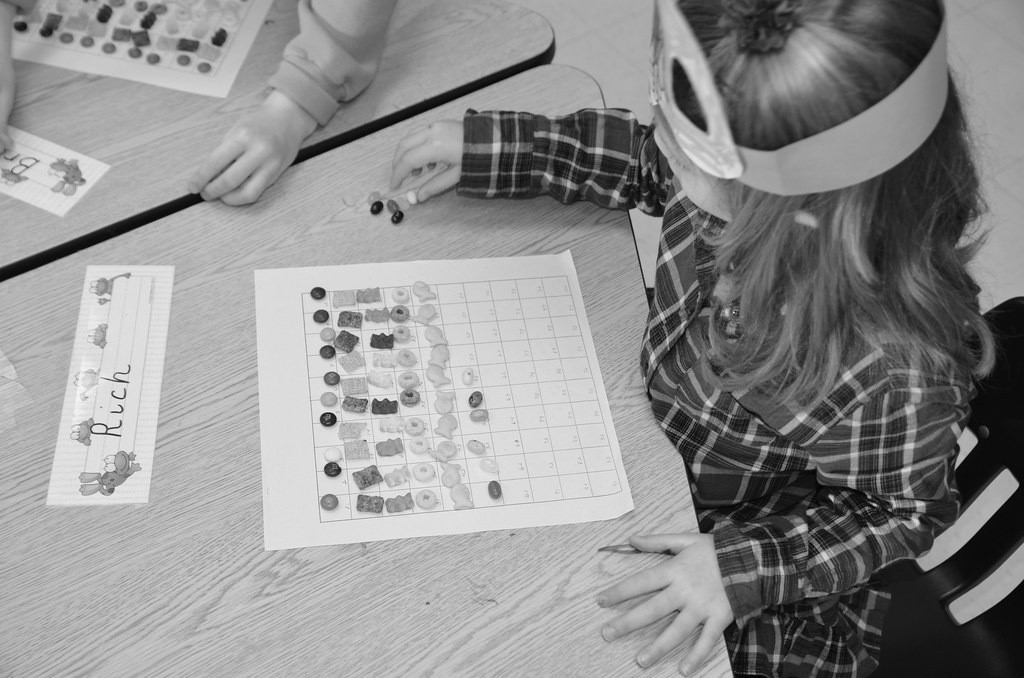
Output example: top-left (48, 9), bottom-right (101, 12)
top-left (0, 1), bottom-right (559, 274)
top-left (0, 63), bottom-right (732, 677)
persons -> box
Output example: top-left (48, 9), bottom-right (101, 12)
top-left (376, 0), bottom-right (994, 678)
top-left (0, 0), bottom-right (397, 207)
